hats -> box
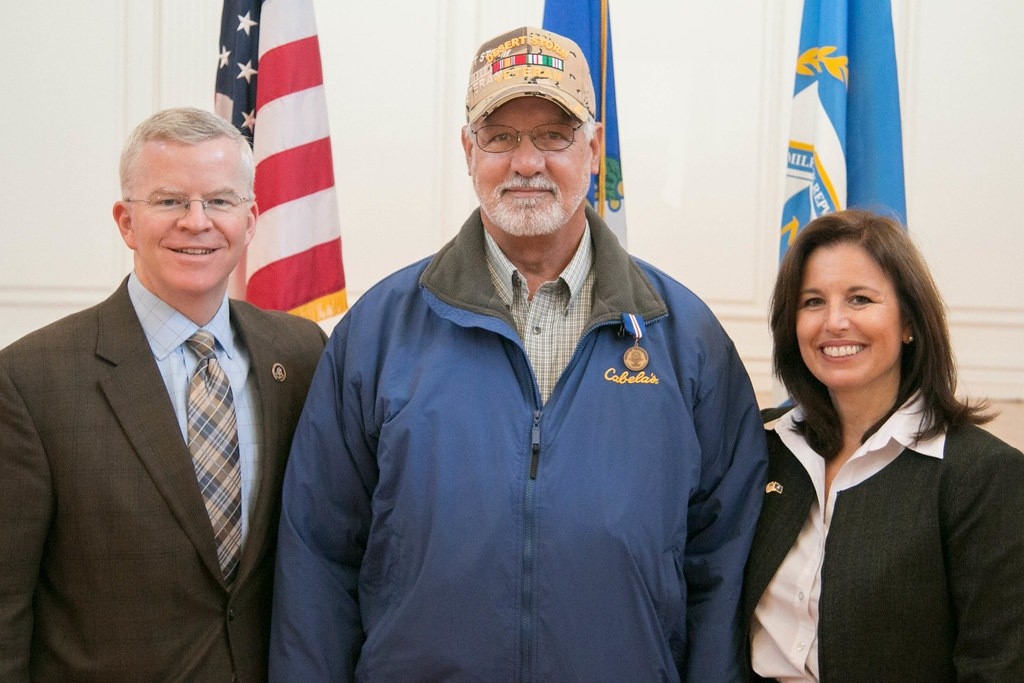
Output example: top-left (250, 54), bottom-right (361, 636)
top-left (463, 27), bottom-right (596, 130)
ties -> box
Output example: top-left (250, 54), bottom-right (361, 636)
top-left (181, 331), bottom-right (244, 580)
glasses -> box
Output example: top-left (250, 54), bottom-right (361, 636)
top-left (466, 121), bottom-right (588, 154)
top-left (121, 193), bottom-right (254, 218)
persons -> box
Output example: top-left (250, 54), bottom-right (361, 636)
top-left (1, 105), bottom-right (330, 682)
top-left (267, 43), bottom-right (768, 682)
top-left (736, 206), bottom-right (1023, 681)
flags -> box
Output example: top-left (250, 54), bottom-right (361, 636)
top-left (779, 1), bottom-right (908, 274)
top-left (215, 0), bottom-right (351, 337)
top-left (540, 0), bottom-right (626, 262)
